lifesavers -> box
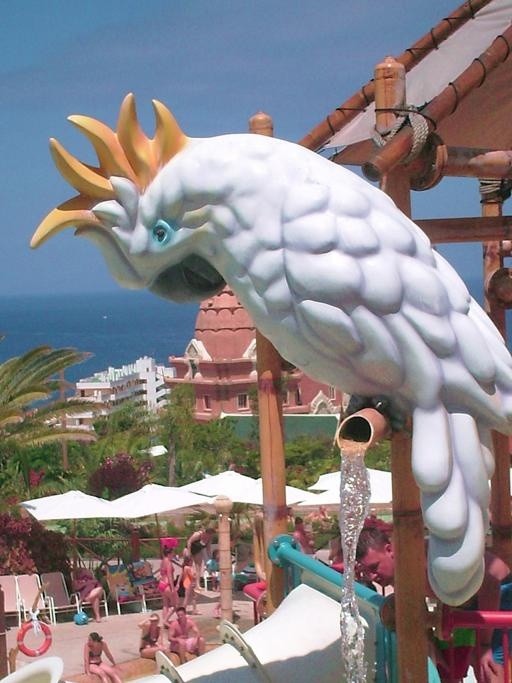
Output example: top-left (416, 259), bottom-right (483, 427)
top-left (16, 620), bottom-right (52, 656)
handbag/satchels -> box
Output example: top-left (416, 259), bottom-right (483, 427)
top-left (74, 612), bottom-right (88, 625)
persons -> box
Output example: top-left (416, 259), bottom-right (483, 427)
top-left (71, 528), bottom-right (236, 683)
top-left (285, 504), bottom-right (512, 683)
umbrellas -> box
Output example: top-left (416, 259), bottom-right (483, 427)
top-left (178, 463), bottom-right (400, 538)
top-left (108, 482), bottom-right (217, 561)
top-left (17, 490), bottom-right (133, 580)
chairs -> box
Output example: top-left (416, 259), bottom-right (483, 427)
top-left (1, 542), bottom-right (268, 630)
